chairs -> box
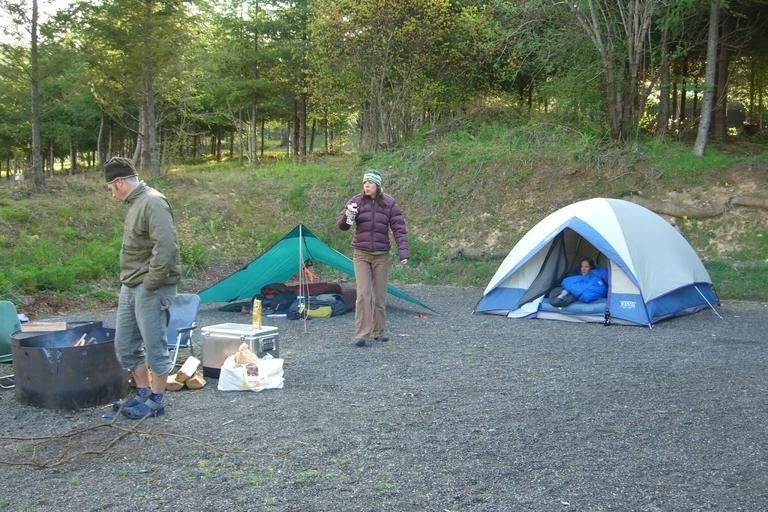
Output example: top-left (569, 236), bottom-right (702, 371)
top-left (166, 294), bottom-right (201, 373)
top-left (0, 300), bottom-right (24, 389)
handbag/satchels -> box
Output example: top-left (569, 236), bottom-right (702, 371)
top-left (217, 354), bottom-right (284, 392)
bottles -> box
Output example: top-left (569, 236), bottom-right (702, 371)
top-left (603, 308), bottom-right (611, 325)
top-left (298, 304), bottom-right (304, 313)
top-left (346, 202), bottom-right (358, 226)
top-left (252, 299), bottom-right (262, 329)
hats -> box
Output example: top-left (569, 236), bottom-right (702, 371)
top-left (102, 155), bottom-right (138, 186)
top-left (362, 169), bottom-right (383, 191)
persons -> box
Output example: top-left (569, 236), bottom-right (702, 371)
top-left (579, 257), bottom-right (595, 276)
top-left (103, 156), bottom-right (181, 419)
top-left (337, 169), bottom-right (410, 346)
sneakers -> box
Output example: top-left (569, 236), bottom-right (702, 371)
top-left (374, 334), bottom-right (390, 343)
top-left (121, 392), bottom-right (164, 419)
top-left (353, 337), bottom-right (365, 347)
top-left (112, 386), bottom-right (152, 413)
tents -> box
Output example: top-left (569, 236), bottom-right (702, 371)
top-left (471, 197), bottom-right (724, 330)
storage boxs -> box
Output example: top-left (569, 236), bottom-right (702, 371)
top-left (200, 323), bottom-right (278, 379)
